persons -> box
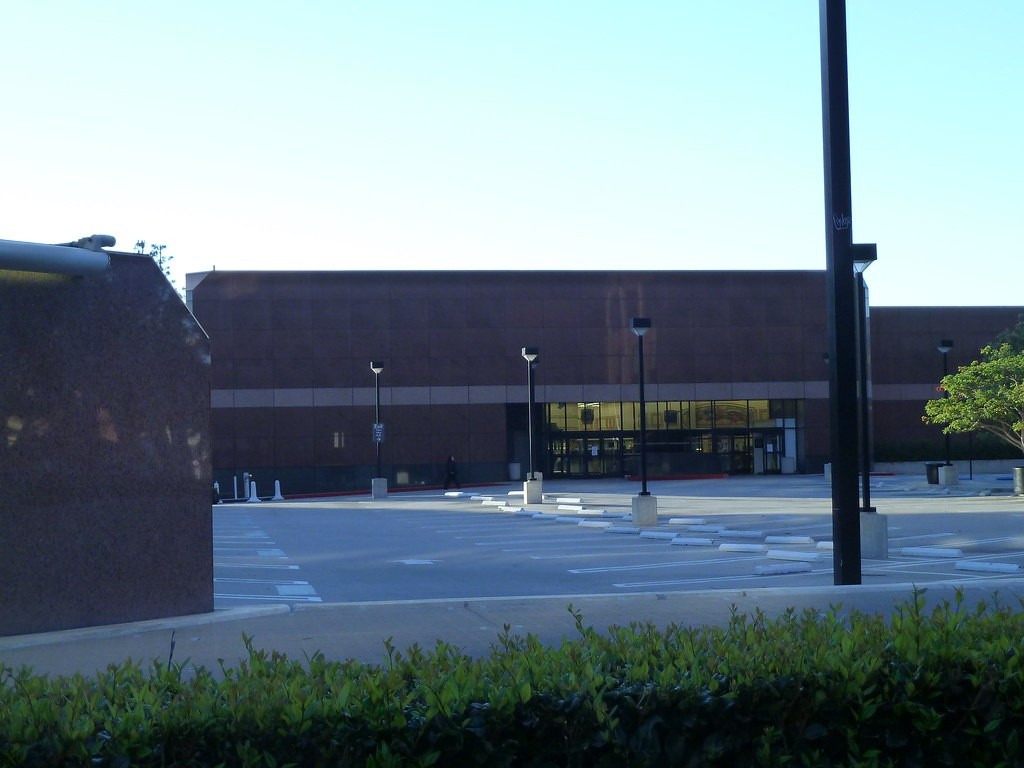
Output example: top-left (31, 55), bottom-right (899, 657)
top-left (446, 455), bottom-right (462, 490)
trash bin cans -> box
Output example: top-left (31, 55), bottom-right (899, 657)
top-left (1013, 467), bottom-right (1024, 494)
